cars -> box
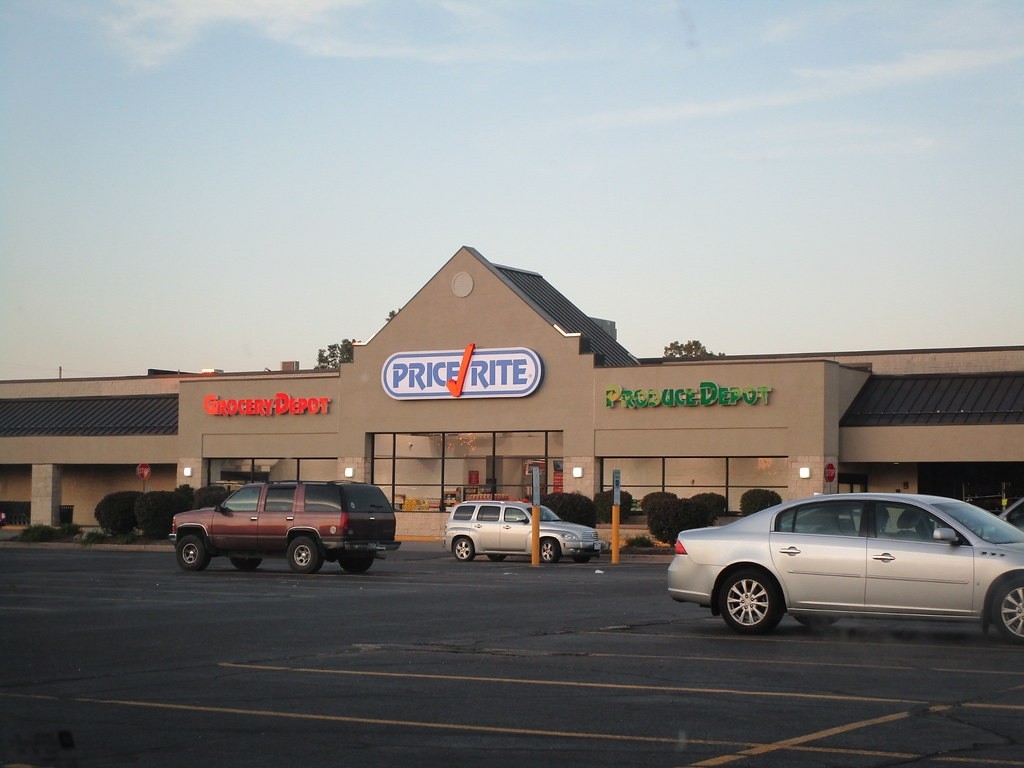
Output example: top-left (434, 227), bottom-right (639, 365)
top-left (666, 493), bottom-right (1024, 643)
top-left (997, 497), bottom-right (1024, 534)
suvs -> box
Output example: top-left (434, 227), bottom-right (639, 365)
top-left (169, 479), bottom-right (402, 574)
top-left (442, 500), bottom-right (602, 564)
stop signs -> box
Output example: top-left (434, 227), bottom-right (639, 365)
top-left (824, 464), bottom-right (836, 482)
top-left (136, 463), bottom-right (151, 480)
